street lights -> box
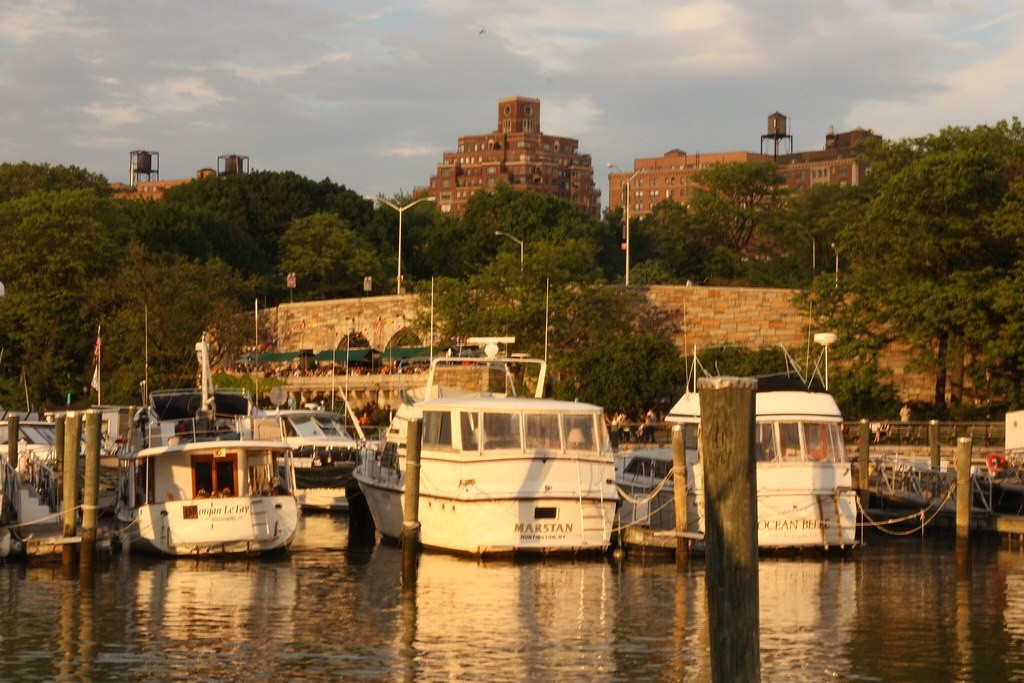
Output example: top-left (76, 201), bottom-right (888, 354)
top-left (495, 231), bottom-right (524, 272)
top-left (607, 163), bottom-right (648, 287)
top-left (365, 195), bottom-right (436, 296)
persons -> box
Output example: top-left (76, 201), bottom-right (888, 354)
top-left (604, 406), bottom-right (659, 453)
top-left (873, 418), bottom-right (890, 444)
top-left (174, 420), bottom-right (189, 439)
top-left (357, 401), bottom-right (391, 424)
top-left (899, 403), bottom-right (912, 423)
top-left (218, 360), bottom-right (476, 377)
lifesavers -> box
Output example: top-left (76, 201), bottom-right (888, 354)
top-left (985, 451), bottom-right (1007, 474)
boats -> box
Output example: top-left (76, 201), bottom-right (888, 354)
top-left (616, 296), bottom-right (861, 553)
top-left (1, 298), bottom-right (377, 561)
top-left (351, 276), bottom-right (619, 554)
